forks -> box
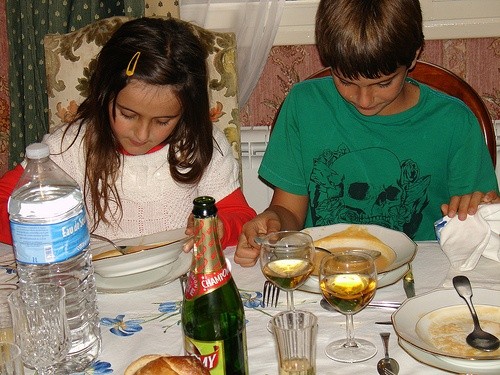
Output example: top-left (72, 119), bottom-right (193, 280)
top-left (263, 280), bottom-right (280, 308)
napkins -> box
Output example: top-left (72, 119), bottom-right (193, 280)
top-left (440, 202), bottom-right (500, 271)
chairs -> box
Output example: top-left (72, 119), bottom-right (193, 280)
top-left (44, 16), bottom-right (242, 195)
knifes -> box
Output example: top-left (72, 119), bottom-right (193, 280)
top-left (403, 271), bottom-right (415, 298)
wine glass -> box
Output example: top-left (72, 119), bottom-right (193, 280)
top-left (260, 231), bottom-right (318, 336)
top-left (9, 282), bottom-right (73, 375)
top-left (319, 251), bottom-right (378, 363)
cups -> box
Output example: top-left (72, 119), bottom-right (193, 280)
top-left (269, 308), bottom-right (319, 375)
top-left (0, 343), bottom-right (24, 375)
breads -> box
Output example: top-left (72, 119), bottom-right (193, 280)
top-left (123, 353), bottom-right (211, 375)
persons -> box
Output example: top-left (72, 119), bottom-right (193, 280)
top-left (0, 17), bottom-right (262, 255)
top-left (232, 0), bottom-right (500, 267)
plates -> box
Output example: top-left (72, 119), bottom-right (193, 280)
top-left (93, 235), bottom-right (194, 277)
top-left (391, 286), bottom-right (500, 360)
top-left (274, 222), bottom-right (420, 274)
top-left (397, 335), bottom-right (500, 375)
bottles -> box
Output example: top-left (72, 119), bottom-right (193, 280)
top-left (180, 195), bottom-right (251, 375)
top-left (7, 143), bottom-right (103, 373)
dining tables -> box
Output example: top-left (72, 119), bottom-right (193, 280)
top-left (0, 239), bottom-right (500, 375)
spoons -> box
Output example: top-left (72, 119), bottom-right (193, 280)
top-left (377, 332), bottom-right (399, 375)
top-left (452, 274), bottom-right (500, 352)
top-left (90, 234), bottom-right (130, 256)
top-left (256, 236), bottom-right (381, 262)
